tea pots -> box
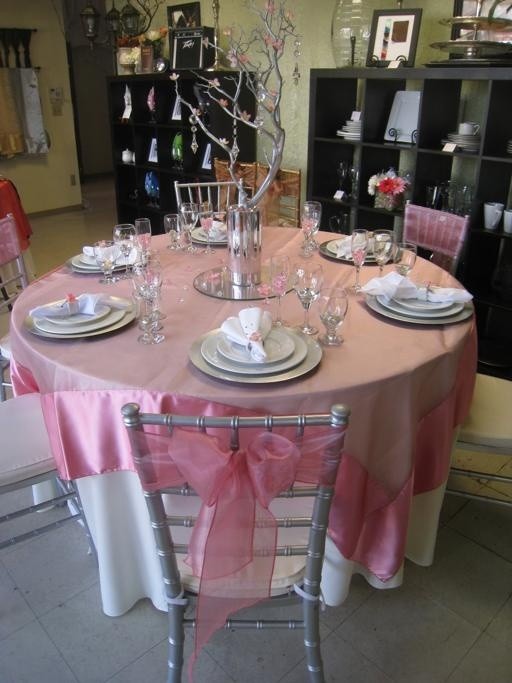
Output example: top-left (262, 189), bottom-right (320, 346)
top-left (122, 148), bottom-right (133, 163)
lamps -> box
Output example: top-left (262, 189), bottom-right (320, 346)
top-left (79, 0), bottom-right (147, 51)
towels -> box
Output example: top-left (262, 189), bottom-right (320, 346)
top-left (356, 271), bottom-right (473, 307)
top-left (0, 67), bottom-right (50, 159)
top-left (220, 306), bottom-right (274, 361)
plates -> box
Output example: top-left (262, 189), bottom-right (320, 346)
top-left (384, 90), bottom-right (420, 145)
top-left (365, 293), bottom-right (475, 325)
top-left (441, 134), bottom-right (479, 151)
top-left (319, 238), bottom-right (404, 264)
top-left (190, 227), bottom-right (229, 247)
top-left (22, 294), bottom-right (135, 340)
top-left (336, 120), bottom-right (361, 140)
top-left (188, 326), bottom-right (323, 384)
top-left (68, 242), bottom-right (148, 273)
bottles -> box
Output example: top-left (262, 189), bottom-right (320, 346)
top-left (336, 162), bottom-right (346, 192)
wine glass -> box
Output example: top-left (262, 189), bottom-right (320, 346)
top-left (133, 260), bottom-right (170, 344)
top-left (262, 254), bottom-right (348, 346)
top-left (349, 229), bottom-right (417, 289)
top-left (164, 202), bottom-right (214, 255)
top-left (299, 200), bottom-right (322, 257)
top-left (93, 217), bottom-right (151, 284)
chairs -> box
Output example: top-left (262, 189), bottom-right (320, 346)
top-left (173, 157), bottom-right (470, 276)
top-left (121, 400), bottom-right (350, 682)
top-left (444, 372), bottom-right (512, 508)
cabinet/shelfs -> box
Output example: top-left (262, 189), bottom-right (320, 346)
top-left (306, 67), bottom-right (512, 382)
top-left (107, 71), bottom-right (257, 237)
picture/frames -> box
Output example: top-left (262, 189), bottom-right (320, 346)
top-left (449, 0), bottom-right (512, 64)
top-left (366, 9), bottom-right (422, 67)
top-left (167, 2), bottom-right (200, 55)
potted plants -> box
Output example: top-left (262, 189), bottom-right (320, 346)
top-left (118, 36), bottom-right (138, 65)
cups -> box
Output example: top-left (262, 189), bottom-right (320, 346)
top-left (484, 202), bottom-right (512, 234)
top-left (426, 181), bottom-right (475, 216)
top-left (458, 121), bottom-right (479, 134)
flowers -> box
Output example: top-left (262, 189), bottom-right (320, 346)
top-left (139, 26), bottom-right (168, 58)
